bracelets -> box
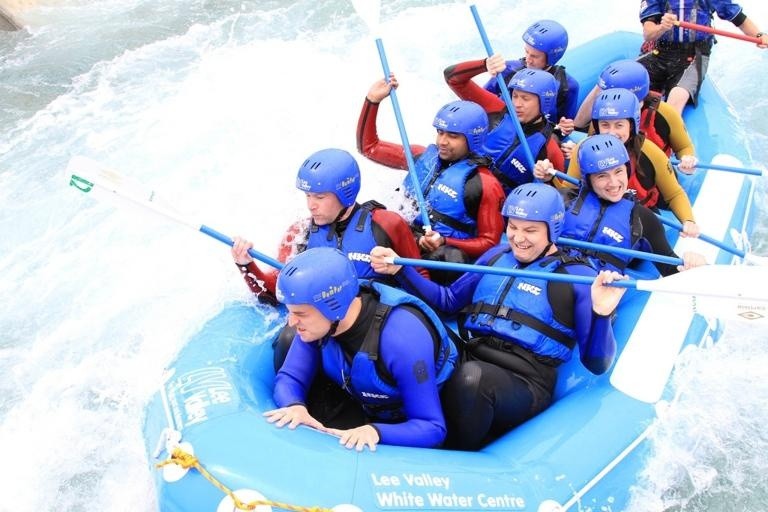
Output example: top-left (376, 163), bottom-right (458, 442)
top-left (758, 32), bottom-right (765, 37)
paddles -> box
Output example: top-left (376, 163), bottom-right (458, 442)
top-left (351, 0), bottom-right (441, 245)
top-left (382, 257), bottom-right (768, 321)
top-left (68, 154), bottom-right (287, 272)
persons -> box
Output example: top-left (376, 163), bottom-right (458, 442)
top-left (231, 18), bottom-right (700, 452)
top-left (638, 0), bottom-right (766, 117)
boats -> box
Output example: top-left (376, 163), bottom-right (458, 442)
top-left (142, 29), bottom-right (757, 511)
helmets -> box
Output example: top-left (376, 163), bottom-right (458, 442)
top-left (522, 20), bottom-right (569, 66)
top-left (577, 134), bottom-right (630, 176)
top-left (598, 59), bottom-right (649, 102)
top-left (433, 101), bottom-right (489, 153)
top-left (592, 88), bottom-right (640, 136)
top-left (508, 68), bottom-right (555, 116)
top-left (275, 246), bottom-right (360, 323)
top-left (293, 149), bottom-right (362, 207)
top-left (502, 183), bottom-right (566, 243)
